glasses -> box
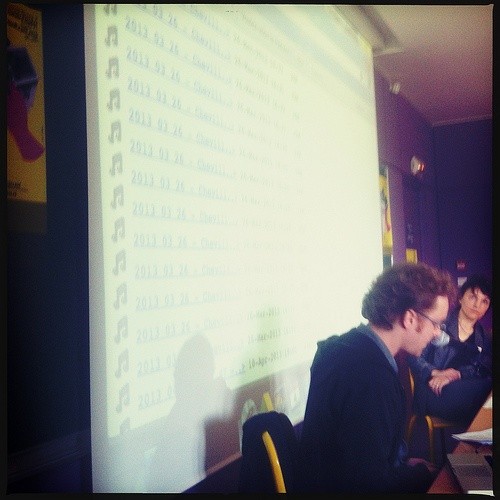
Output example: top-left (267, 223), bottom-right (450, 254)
top-left (413, 310), bottom-right (446, 331)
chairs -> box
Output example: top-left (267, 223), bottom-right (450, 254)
top-left (256, 390), bottom-right (294, 499)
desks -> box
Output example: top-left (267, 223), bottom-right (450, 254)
top-left (429, 389), bottom-right (494, 495)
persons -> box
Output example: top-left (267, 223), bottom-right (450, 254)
top-left (407, 277), bottom-right (496, 457)
top-left (299, 256), bottom-right (463, 499)
